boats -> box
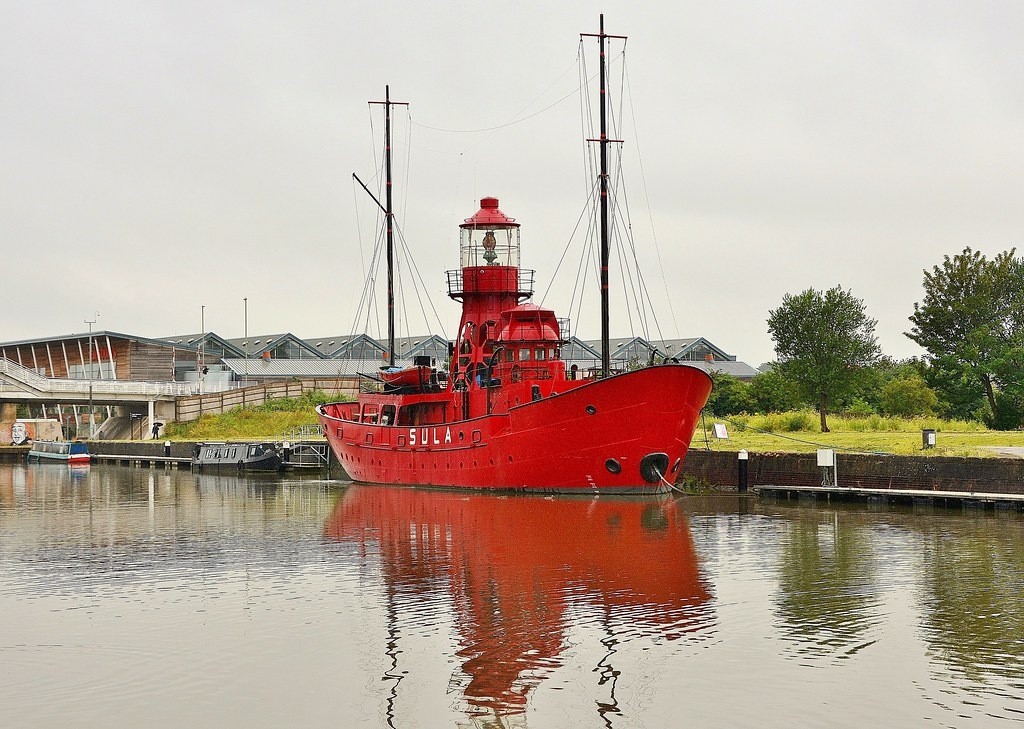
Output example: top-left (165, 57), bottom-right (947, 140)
top-left (192, 443), bottom-right (283, 473)
top-left (27, 439), bottom-right (91, 463)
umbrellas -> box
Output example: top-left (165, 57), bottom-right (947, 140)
top-left (195, 443), bottom-right (203, 446)
top-left (153, 422), bottom-right (163, 427)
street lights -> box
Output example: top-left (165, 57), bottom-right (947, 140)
top-left (243, 297), bottom-right (247, 388)
top-left (201, 305), bottom-right (205, 366)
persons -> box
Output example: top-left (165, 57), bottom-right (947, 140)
top-left (193, 442), bottom-right (203, 460)
top-left (152, 424), bottom-right (159, 440)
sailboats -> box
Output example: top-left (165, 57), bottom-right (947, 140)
top-left (314, 13), bottom-right (715, 493)
